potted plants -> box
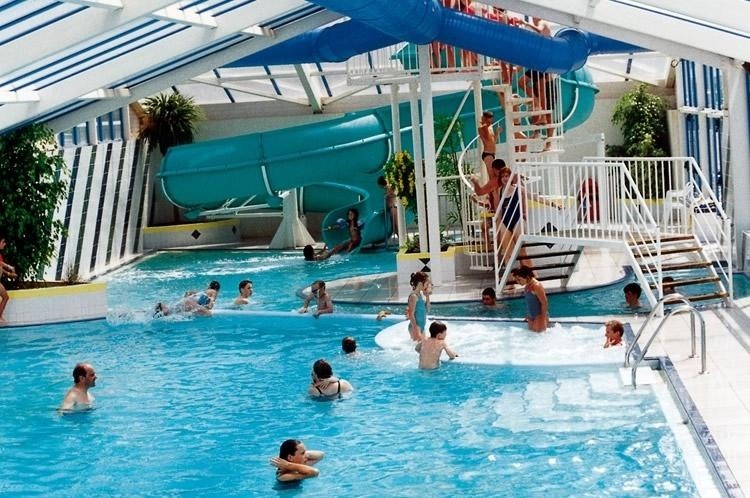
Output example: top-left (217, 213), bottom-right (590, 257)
top-left (136, 91), bottom-right (207, 156)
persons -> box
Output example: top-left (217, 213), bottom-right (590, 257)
top-left (431, 0), bottom-right (558, 151)
top-left (621, 283), bottom-right (643, 308)
top-left (477, 110), bottom-right (502, 181)
top-left (268, 438), bottom-right (323, 486)
top-left (331, 207), bottom-right (368, 255)
top-left (57, 362), bottom-right (101, 412)
top-left (377, 306), bottom-right (411, 321)
top-left (501, 94), bottom-right (533, 182)
top-left (153, 280), bottom-right (223, 323)
top-left (0, 234), bottom-right (20, 326)
top-left (413, 320), bottom-right (460, 370)
top-left (299, 278), bottom-right (334, 320)
top-left (308, 354), bottom-right (354, 402)
top-left (657, 276), bottom-right (678, 295)
top-left (512, 265), bottom-right (549, 332)
top-left (340, 335), bottom-right (365, 357)
top-left (299, 239), bottom-right (341, 263)
top-left (603, 319), bottom-right (625, 349)
top-left (231, 278), bottom-right (257, 308)
top-left (377, 175), bottom-right (399, 238)
top-left (479, 287), bottom-right (504, 310)
top-left (405, 271), bottom-right (434, 341)
top-left (470, 159), bottom-right (562, 285)
top-left (309, 370), bottom-right (341, 387)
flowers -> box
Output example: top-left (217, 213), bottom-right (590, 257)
top-left (384, 149), bottom-right (443, 253)
